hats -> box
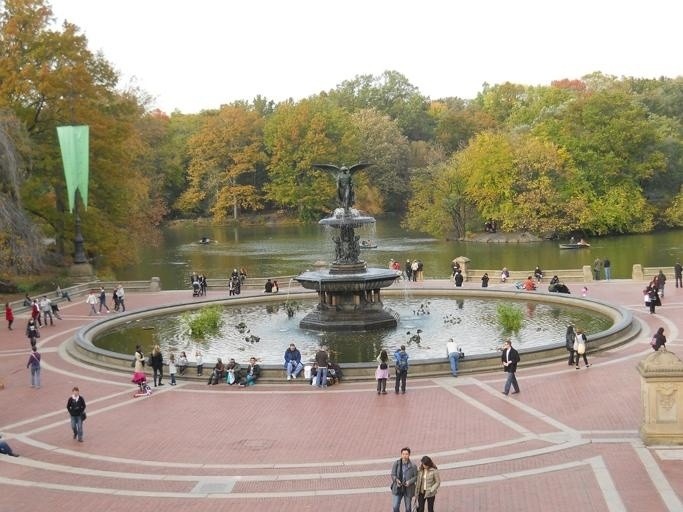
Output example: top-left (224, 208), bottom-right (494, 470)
top-left (136, 343), bottom-right (144, 351)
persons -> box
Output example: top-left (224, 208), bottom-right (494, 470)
top-left (414, 456), bottom-right (440, 512)
top-left (658, 271), bottom-right (666, 297)
top-left (410, 260), bottom-right (418, 281)
top-left (38, 297), bottom-right (42, 311)
top-left (200, 273), bottom-right (207, 296)
top-left (67, 387), bottom-right (86, 442)
top-left (97, 286), bottom-right (110, 313)
top-left (652, 327), bottom-right (666, 351)
top-left (116, 285), bottom-right (125, 312)
top-left (501, 267), bottom-right (507, 281)
top-left (556, 283), bottom-right (568, 293)
top-left (232, 269), bottom-right (239, 281)
top-left (550, 275), bottom-right (560, 284)
top-left (375, 350), bottom-right (393, 395)
top-left (569, 237), bottom-right (577, 244)
top-left (208, 358), bottom-right (225, 385)
top-left (566, 326), bottom-right (575, 365)
top-left (26, 346), bottom-right (41, 389)
top-left (5, 301), bottom-right (14, 330)
top-left (240, 267), bottom-right (246, 284)
top-left (675, 261), bottom-right (683, 288)
top-left (405, 262), bottom-right (412, 282)
top-left (167, 354), bottom-right (176, 385)
top-left (502, 341), bottom-right (520, 396)
top-left (393, 262), bottom-right (400, 283)
top-left (272, 281), bottom-right (278, 292)
top-left (113, 287), bottom-right (118, 310)
top-left (451, 259), bottom-right (460, 277)
top-left (234, 277), bottom-right (240, 294)
top-left (594, 256), bottom-right (602, 280)
top-left (548, 285), bottom-right (557, 292)
top-left (481, 273), bottom-right (489, 287)
top-left (239, 358), bottom-right (260, 386)
top-left (653, 276), bottom-right (658, 290)
top-left (394, 345), bottom-right (409, 394)
top-left (574, 329), bottom-right (589, 369)
top-left (56, 287), bottom-right (71, 302)
top-left (178, 351), bottom-right (187, 376)
top-left (135, 344), bottom-right (145, 390)
top-left (526, 276), bottom-right (537, 290)
top-left (391, 447), bottom-right (418, 512)
top-left (604, 256), bottom-right (611, 280)
top-left (195, 351), bottom-right (203, 376)
top-left (41, 296), bottom-right (53, 325)
top-left (152, 345), bottom-right (164, 387)
top-left (32, 300), bottom-right (42, 328)
top-left (24, 293), bottom-right (33, 306)
top-left (455, 270), bottom-right (463, 287)
top-left (645, 281), bottom-right (661, 314)
top-left (86, 292), bottom-right (98, 316)
top-left (228, 278), bottom-right (234, 296)
top-left (388, 258), bottom-right (395, 270)
top-left (191, 272), bottom-right (199, 284)
top-left (284, 344), bottom-right (303, 381)
top-left (0, 436), bottom-right (20, 457)
top-left (265, 280), bottom-right (272, 292)
top-left (26, 317), bottom-right (38, 347)
top-left (311, 345), bottom-right (342, 388)
top-left (447, 339), bottom-right (459, 377)
top-left (417, 259), bottom-right (423, 272)
top-left (223, 358), bottom-right (242, 384)
top-left (534, 265), bottom-right (543, 284)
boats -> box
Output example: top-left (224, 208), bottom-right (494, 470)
top-left (559, 243), bottom-right (588, 249)
top-left (199, 237), bottom-right (210, 244)
top-left (558, 241), bottom-right (591, 250)
top-left (200, 238), bottom-right (210, 244)
top-left (360, 242), bottom-right (377, 249)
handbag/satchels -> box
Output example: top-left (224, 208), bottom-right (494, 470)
top-left (36, 331), bottom-right (41, 338)
top-left (650, 337), bottom-right (657, 345)
top-left (390, 460), bottom-right (399, 495)
top-left (411, 497), bottom-right (419, 512)
top-left (81, 411), bottom-right (87, 421)
top-left (644, 294), bottom-right (651, 302)
top-left (575, 336), bottom-right (586, 354)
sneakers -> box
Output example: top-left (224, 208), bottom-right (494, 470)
top-left (586, 363), bottom-right (593, 368)
top-left (377, 391), bottom-right (381, 396)
top-left (37, 385), bottom-right (41, 390)
top-left (8, 453), bottom-right (20, 458)
top-left (97, 311), bottom-right (103, 314)
top-left (502, 391), bottom-right (510, 396)
top-left (291, 373), bottom-right (297, 379)
top-left (79, 439), bottom-right (84, 443)
top-left (512, 391), bottom-right (519, 394)
top-left (382, 391), bottom-right (388, 396)
top-left (158, 384), bottom-right (164, 386)
top-left (73, 435), bottom-right (78, 440)
top-left (197, 372), bottom-right (202, 376)
top-left (107, 310), bottom-right (112, 314)
top-left (30, 383), bottom-right (35, 388)
top-left (576, 366), bottom-right (580, 369)
top-left (287, 375), bottom-right (291, 380)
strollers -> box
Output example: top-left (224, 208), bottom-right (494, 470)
top-left (192, 281), bottom-right (204, 298)
